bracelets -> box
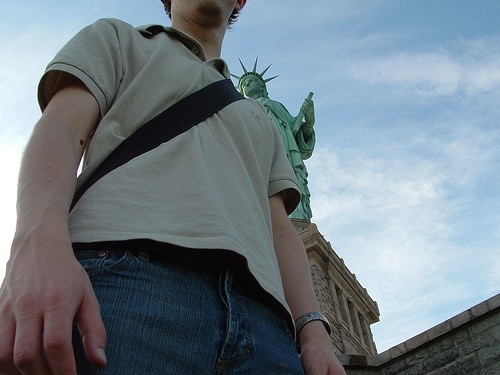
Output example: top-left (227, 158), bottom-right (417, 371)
top-left (294, 310), bottom-right (331, 354)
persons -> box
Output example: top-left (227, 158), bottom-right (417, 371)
top-left (2, 0), bottom-right (346, 375)
top-left (230, 54), bottom-right (316, 221)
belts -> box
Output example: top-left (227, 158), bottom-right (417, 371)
top-left (72, 243), bottom-right (259, 293)
